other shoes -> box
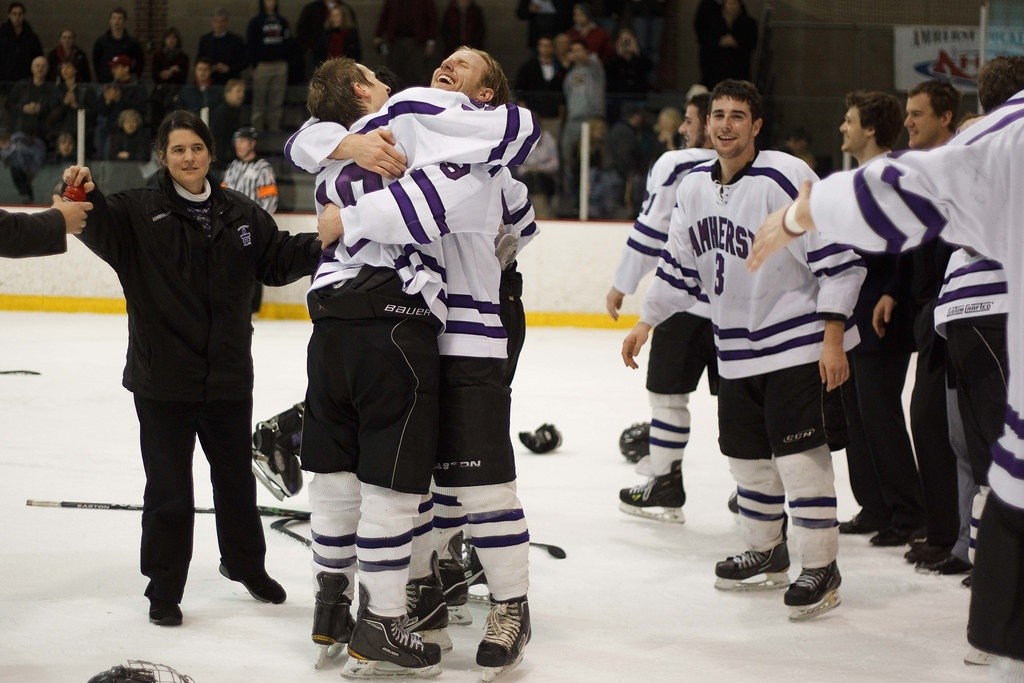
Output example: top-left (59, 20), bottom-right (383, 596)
top-left (837, 504), bottom-right (879, 534)
top-left (869, 524), bottom-right (916, 548)
top-left (902, 541), bottom-right (975, 588)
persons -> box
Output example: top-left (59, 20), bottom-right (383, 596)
top-left (60, 111), bottom-right (337, 626)
top-left (372, 0), bottom-right (488, 86)
top-left (251, 43), bottom-right (544, 683)
top-left (222, 127), bottom-right (279, 216)
top-left (0, 194), bottom-right (94, 259)
top-left (605, 56), bottom-right (1024, 672)
top-left (512, 0), bottom-right (829, 222)
top-left (147, 0), bottom-right (363, 186)
top-left (0, 0), bottom-right (152, 204)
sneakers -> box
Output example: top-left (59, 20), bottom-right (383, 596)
top-left (341, 583), bottom-right (442, 681)
top-left (149, 595), bottom-right (183, 626)
top-left (399, 549), bottom-right (452, 650)
top-left (964, 618), bottom-right (1024, 673)
top-left (619, 458), bottom-right (686, 523)
top-left (714, 509), bottom-right (792, 593)
top-left (311, 571), bottom-right (356, 669)
top-left (441, 526), bottom-right (466, 562)
top-left (784, 558), bottom-right (841, 623)
top-left (219, 557), bottom-right (286, 605)
top-left (460, 538), bottom-right (491, 604)
top-left (475, 591), bottom-right (532, 682)
top-left (438, 530), bottom-right (472, 625)
top-left (250, 402), bottom-right (305, 500)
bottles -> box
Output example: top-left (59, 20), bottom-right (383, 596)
top-left (61, 178), bottom-right (87, 203)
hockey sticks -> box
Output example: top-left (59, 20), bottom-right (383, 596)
top-left (268, 518), bottom-right (313, 547)
top-left (26, 499), bottom-right (311, 519)
top-left (0, 370), bottom-right (42, 375)
top-left (530, 541), bottom-right (567, 559)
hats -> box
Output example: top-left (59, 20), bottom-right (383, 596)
top-left (109, 55), bottom-right (130, 66)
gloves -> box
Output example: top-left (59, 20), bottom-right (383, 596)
top-left (620, 422), bottom-right (650, 463)
top-left (519, 423), bottom-right (562, 453)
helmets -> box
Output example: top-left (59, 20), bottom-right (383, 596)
top-left (233, 127), bottom-right (259, 140)
top-left (85, 665), bottom-right (156, 683)
